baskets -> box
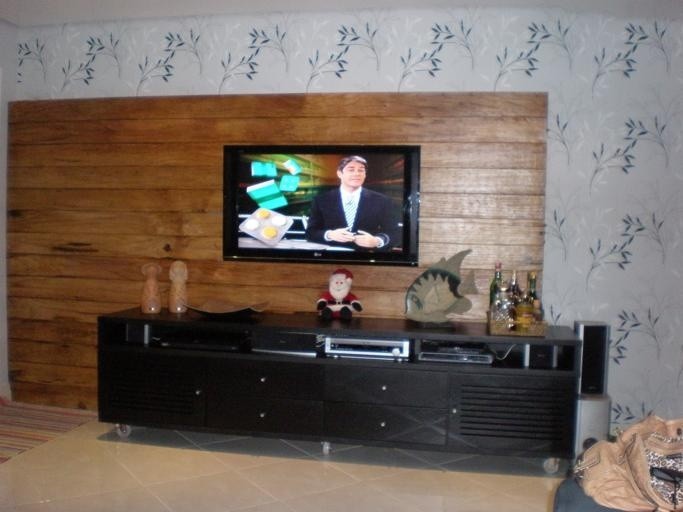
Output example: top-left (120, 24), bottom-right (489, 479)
top-left (486, 311), bottom-right (547, 338)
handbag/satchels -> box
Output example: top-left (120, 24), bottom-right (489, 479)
top-left (573, 416), bottom-right (683, 511)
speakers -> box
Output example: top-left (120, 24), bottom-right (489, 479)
top-left (575, 398), bottom-right (613, 463)
top-left (578, 318), bottom-right (611, 396)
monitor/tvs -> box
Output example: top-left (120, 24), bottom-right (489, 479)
top-left (221, 142), bottom-right (423, 269)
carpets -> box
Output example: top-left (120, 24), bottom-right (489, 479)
top-left (1, 399), bottom-right (98, 464)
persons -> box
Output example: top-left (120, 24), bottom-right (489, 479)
top-left (305, 154), bottom-right (404, 254)
top-left (313, 267), bottom-right (363, 323)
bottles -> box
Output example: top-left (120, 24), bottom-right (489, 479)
top-left (489, 260), bottom-right (545, 331)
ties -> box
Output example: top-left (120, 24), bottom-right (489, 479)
top-left (344, 197), bottom-right (356, 226)
